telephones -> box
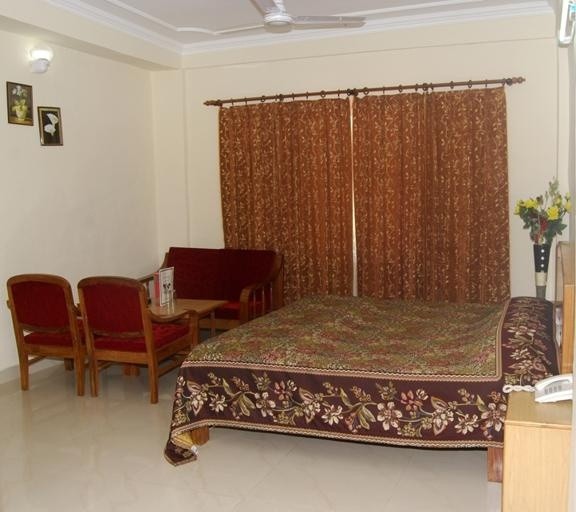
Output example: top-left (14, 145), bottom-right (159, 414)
top-left (503, 371), bottom-right (575, 407)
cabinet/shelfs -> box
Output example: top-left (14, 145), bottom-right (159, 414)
top-left (503, 388), bottom-right (573, 511)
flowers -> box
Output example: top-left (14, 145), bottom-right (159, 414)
top-left (514, 178), bottom-right (569, 243)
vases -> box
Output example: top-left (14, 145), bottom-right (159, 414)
top-left (531, 244), bottom-right (552, 298)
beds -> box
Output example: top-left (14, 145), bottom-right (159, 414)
top-left (164, 295), bottom-right (554, 484)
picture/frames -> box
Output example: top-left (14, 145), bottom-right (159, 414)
top-left (38, 107), bottom-right (62, 147)
top-left (6, 82), bottom-right (33, 126)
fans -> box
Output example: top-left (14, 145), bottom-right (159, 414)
top-left (215, 0), bottom-right (367, 36)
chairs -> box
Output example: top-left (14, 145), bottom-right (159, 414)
top-left (4, 274), bottom-right (85, 395)
top-left (134, 248), bottom-right (284, 338)
top-left (77, 275), bottom-right (192, 403)
